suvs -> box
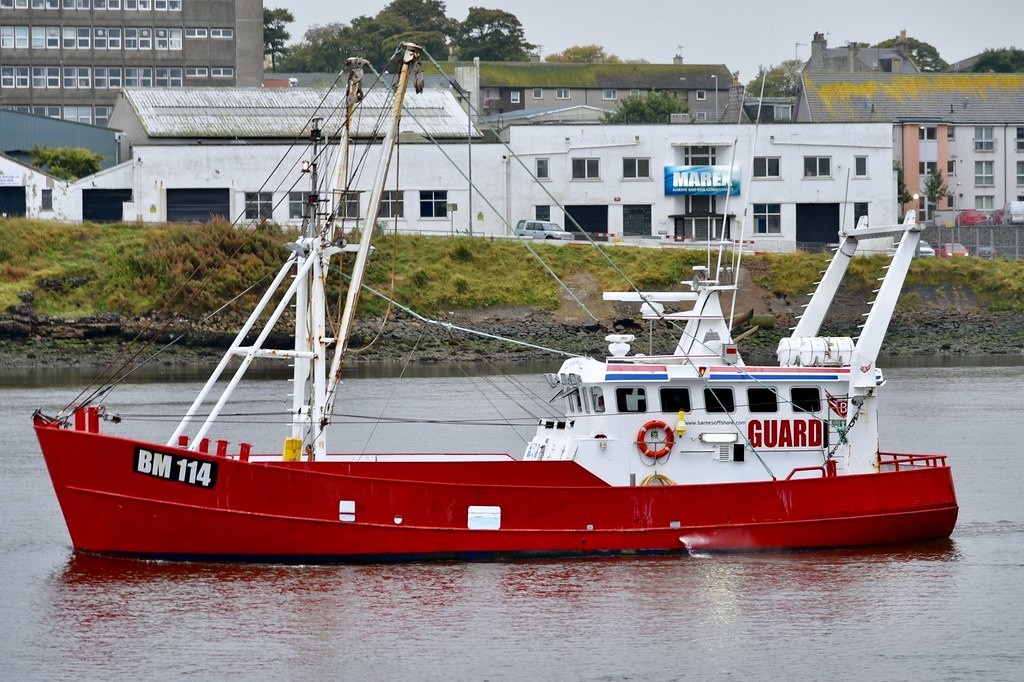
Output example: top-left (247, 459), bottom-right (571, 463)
top-left (514, 219), bottom-right (575, 240)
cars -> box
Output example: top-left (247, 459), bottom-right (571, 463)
top-left (969, 246), bottom-right (998, 260)
top-left (934, 242), bottom-right (970, 257)
top-left (955, 208), bottom-right (988, 226)
top-left (987, 208), bottom-right (1004, 225)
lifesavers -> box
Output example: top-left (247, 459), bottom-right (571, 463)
top-left (636, 420), bottom-right (675, 458)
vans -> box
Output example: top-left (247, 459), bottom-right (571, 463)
top-left (919, 240), bottom-right (935, 257)
top-left (1002, 201), bottom-right (1024, 225)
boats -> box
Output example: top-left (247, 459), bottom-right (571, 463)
top-left (31, 41), bottom-right (962, 570)
